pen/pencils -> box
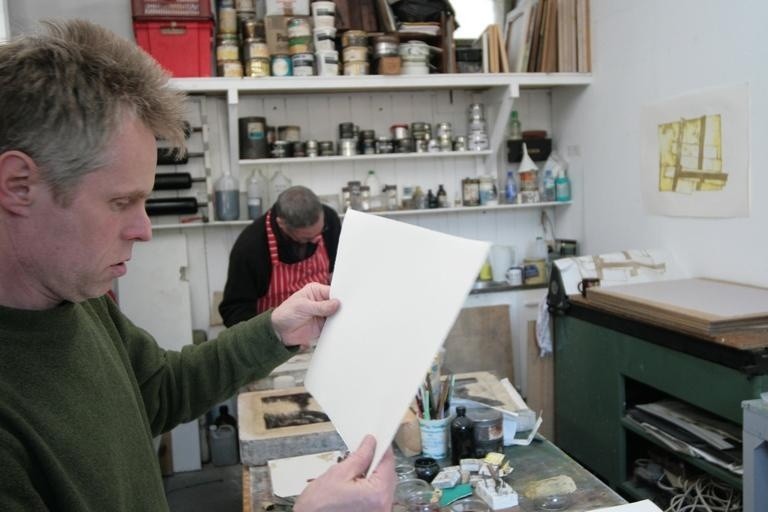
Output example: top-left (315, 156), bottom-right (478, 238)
top-left (409, 370), bottom-right (456, 420)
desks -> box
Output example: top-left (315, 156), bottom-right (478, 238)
top-left (236, 348), bottom-right (629, 511)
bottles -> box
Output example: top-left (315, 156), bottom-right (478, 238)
top-left (450, 407), bottom-right (474, 466)
top-left (466, 408), bottom-right (504, 458)
top-left (215, 405), bottom-right (236, 430)
top-left (215, 142), bottom-right (570, 222)
top-left (235, 103), bottom-right (497, 153)
top-left (215, 0), bottom-right (398, 77)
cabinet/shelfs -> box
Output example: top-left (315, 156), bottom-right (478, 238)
top-left (550, 296), bottom-right (768, 512)
top-left (436, 281), bottom-right (555, 441)
top-left (152, 70), bottom-right (591, 229)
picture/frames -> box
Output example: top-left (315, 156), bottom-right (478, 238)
top-left (638, 82), bottom-right (751, 220)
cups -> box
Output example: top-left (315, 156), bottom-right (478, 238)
top-left (448, 499), bottom-right (490, 512)
top-left (391, 458), bottom-right (442, 512)
top-left (415, 411), bottom-right (451, 459)
top-left (577, 277), bottom-right (600, 298)
top-left (480, 236), bottom-right (576, 286)
top-left (533, 494), bottom-right (572, 512)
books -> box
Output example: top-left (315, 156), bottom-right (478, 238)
top-left (396, 22), bottom-right (441, 36)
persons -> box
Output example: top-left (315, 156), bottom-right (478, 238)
top-left (218, 186), bottom-right (342, 327)
top-left (0, 17), bottom-right (397, 512)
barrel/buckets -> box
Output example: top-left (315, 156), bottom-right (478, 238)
top-left (207, 423), bottom-right (240, 467)
top-left (523, 235), bottom-right (550, 287)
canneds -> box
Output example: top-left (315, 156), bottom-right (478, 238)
top-left (241, 18), bottom-right (270, 76)
top-left (342, 29), bottom-right (370, 75)
top-left (312, 0), bottom-right (340, 75)
top-left (272, 55), bottom-right (292, 75)
top-left (287, 18), bottom-right (314, 75)
top-left (374, 36), bottom-right (399, 56)
top-left (216, 6), bottom-right (243, 77)
top-left (239, 102), bottom-right (488, 159)
